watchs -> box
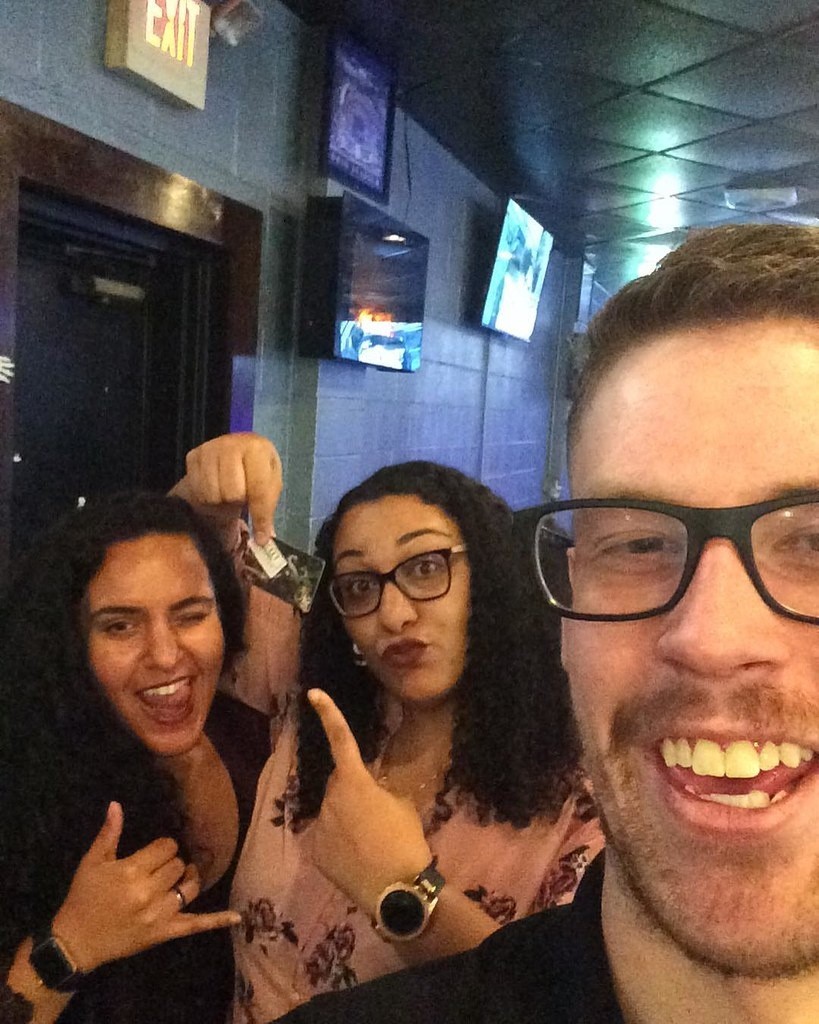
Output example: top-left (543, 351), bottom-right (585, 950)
top-left (370, 864), bottom-right (448, 944)
top-left (26, 921), bottom-right (87, 996)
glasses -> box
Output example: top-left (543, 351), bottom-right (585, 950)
top-left (326, 543), bottom-right (469, 618)
top-left (511, 493), bottom-right (819, 623)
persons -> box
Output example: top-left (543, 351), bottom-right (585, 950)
top-left (0, 222), bottom-right (819, 1024)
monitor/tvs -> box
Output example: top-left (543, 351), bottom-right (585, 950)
top-left (476, 196), bottom-right (556, 341)
top-left (328, 189), bottom-right (429, 375)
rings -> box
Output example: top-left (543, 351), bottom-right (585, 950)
top-left (173, 886), bottom-right (188, 912)
